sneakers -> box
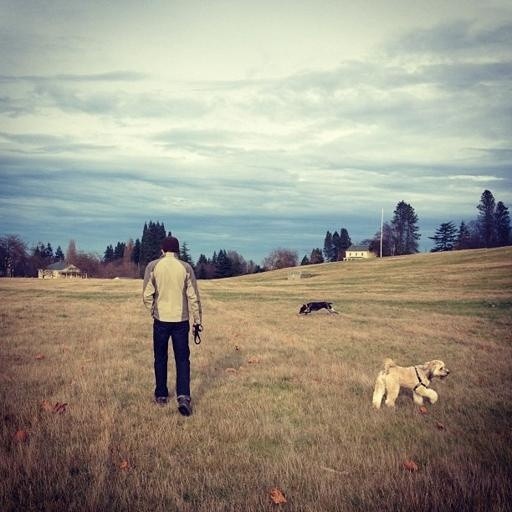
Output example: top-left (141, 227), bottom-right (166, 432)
top-left (176, 395), bottom-right (192, 416)
top-left (154, 396), bottom-right (167, 405)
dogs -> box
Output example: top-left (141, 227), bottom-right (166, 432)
top-left (298, 301), bottom-right (339, 316)
top-left (372, 357), bottom-right (450, 411)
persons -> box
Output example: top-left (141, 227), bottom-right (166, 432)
top-left (141, 236), bottom-right (205, 416)
